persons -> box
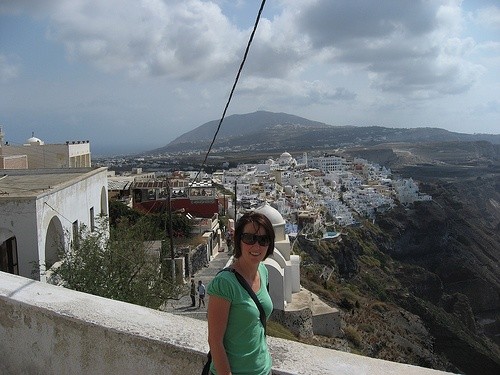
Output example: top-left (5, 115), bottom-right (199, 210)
top-left (190, 278), bottom-right (198, 306)
top-left (206, 211), bottom-right (275, 375)
top-left (224, 229), bottom-right (235, 255)
top-left (196, 280), bottom-right (207, 310)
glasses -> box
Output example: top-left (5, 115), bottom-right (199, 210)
top-left (241, 233), bottom-right (272, 246)
